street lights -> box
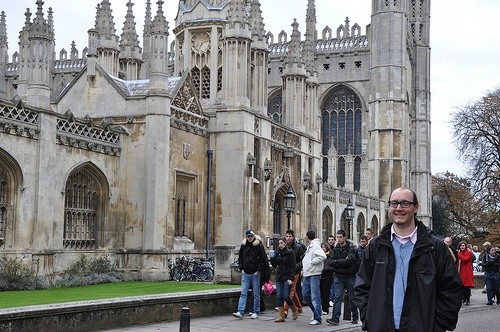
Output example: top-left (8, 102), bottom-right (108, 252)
top-left (282, 185), bottom-right (297, 231)
top-left (344, 198), bottom-right (355, 240)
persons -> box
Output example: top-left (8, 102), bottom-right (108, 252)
top-left (478, 241), bottom-right (500, 305)
top-left (353, 187), bottom-right (464, 332)
top-left (233, 230), bottom-right (270, 319)
top-left (443, 237), bottom-right (478, 305)
top-left (270, 228), bottom-right (375, 324)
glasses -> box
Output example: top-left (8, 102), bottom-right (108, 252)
top-left (387, 199), bottom-right (414, 208)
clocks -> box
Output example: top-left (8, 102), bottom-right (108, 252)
top-left (191, 32), bottom-right (211, 55)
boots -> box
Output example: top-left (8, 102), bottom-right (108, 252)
top-left (290, 303), bottom-right (298, 320)
top-left (274, 307), bottom-right (285, 322)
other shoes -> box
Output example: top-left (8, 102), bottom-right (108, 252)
top-left (309, 320), bottom-right (319, 325)
top-left (486, 302), bottom-right (493, 305)
top-left (232, 311), bottom-right (242, 318)
top-left (251, 314), bottom-right (258, 319)
top-left (329, 301), bottom-right (333, 306)
top-left (284, 311), bottom-right (287, 318)
top-left (327, 319), bottom-right (337, 325)
top-left (323, 312), bottom-right (327, 315)
top-left (482, 290), bottom-right (487, 293)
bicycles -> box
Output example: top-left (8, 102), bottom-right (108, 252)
top-left (168, 255), bottom-right (216, 282)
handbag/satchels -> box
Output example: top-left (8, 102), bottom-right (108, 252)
top-left (263, 280), bottom-right (274, 295)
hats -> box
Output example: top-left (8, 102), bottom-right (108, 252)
top-left (482, 242), bottom-right (491, 246)
top-left (246, 230), bottom-right (254, 237)
